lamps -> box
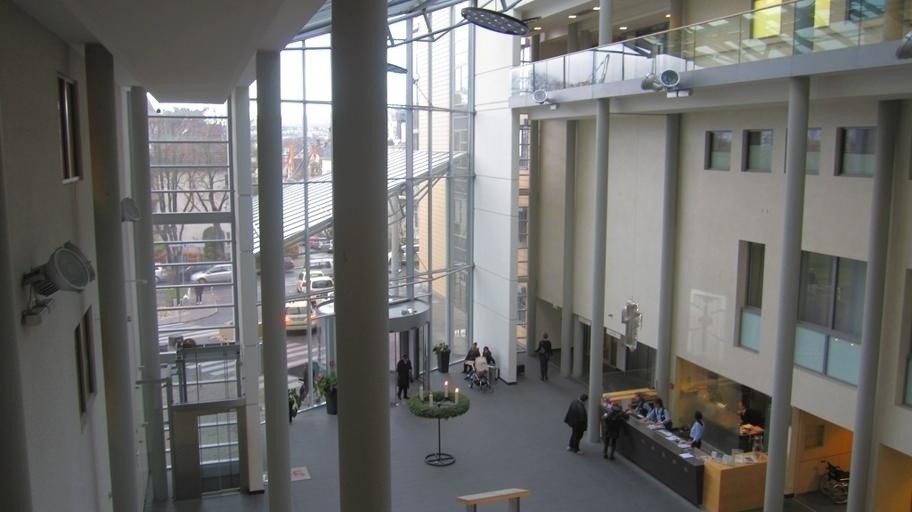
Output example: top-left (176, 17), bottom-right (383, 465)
top-left (116, 193), bottom-right (143, 223)
top-left (530, 88), bottom-right (549, 107)
top-left (20, 238), bottom-right (99, 324)
top-left (894, 28), bottom-right (912, 61)
top-left (641, 68), bottom-right (682, 93)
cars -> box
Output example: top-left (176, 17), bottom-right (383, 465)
top-left (297, 269), bottom-right (323, 294)
top-left (190, 263), bottom-right (234, 284)
top-left (285, 300), bottom-right (318, 332)
top-left (309, 236), bottom-right (420, 274)
top-left (178, 260), bottom-right (211, 280)
top-left (257, 256), bottom-right (295, 277)
top-left (154, 262), bottom-right (168, 283)
top-left (305, 258), bottom-right (333, 279)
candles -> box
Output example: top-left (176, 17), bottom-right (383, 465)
top-left (417, 379), bottom-right (462, 408)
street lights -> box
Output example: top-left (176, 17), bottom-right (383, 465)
top-left (395, 110), bottom-right (414, 301)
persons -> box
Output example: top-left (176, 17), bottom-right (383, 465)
top-left (392, 352), bottom-right (414, 402)
top-left (736, 399), bottom-right (750, 426)
top-left (533, 332), bottom-right (554, 382)
top-left (562, 392), bottom-right (590, 457)
top-left (686, 411), bottom-right (706, 450)
top-left (598, 394), bottom-right (674, 461)
top-left (461, 341), bottom-right (497, 389)
top-left (193, 281), bottom-right (204, 304)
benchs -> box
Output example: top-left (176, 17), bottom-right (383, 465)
top-left (457, 487), bottom-right (529, 510)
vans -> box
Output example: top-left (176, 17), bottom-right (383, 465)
top-left (308, 276), bottom-right (335, 298)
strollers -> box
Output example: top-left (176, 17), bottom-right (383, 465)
top-left (464, 357), bottom-right (493, 394)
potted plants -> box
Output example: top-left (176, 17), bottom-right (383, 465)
top-left (312, 371), bottom-right (339, 415)
top-left (287, 386), bottom-right (303, 424)
top-left (432, 341), bottom-right (451, 373)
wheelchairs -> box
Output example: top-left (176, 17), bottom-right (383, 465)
top-left (818, 460), bottom-right (850, 505)
